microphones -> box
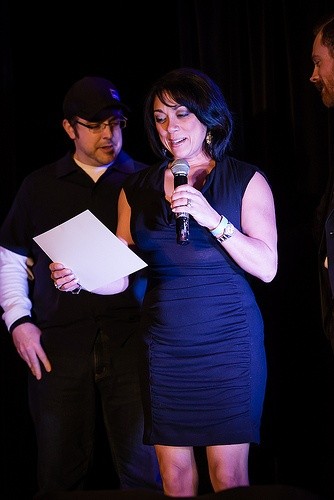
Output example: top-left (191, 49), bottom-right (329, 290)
top-left (170, 159), bottom-right (190, 244)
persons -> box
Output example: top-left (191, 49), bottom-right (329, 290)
top-left (0, 75), bottom-right (164, 500)
top-left (48, 67), bottom-right (279, 498)
top-left (307, 14), bottom-right (334, 353)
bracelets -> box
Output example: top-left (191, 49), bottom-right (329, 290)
top-left (208, 214), bottom-right (235, 244)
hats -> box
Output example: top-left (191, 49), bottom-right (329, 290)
top-left (63, 77), bottom-right (132, 121)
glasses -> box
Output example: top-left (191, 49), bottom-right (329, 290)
top-left (72, 116), bottom-right (128, 133)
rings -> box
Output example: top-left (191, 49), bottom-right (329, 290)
top-left (53, 281), bottom-right (63, 289)
